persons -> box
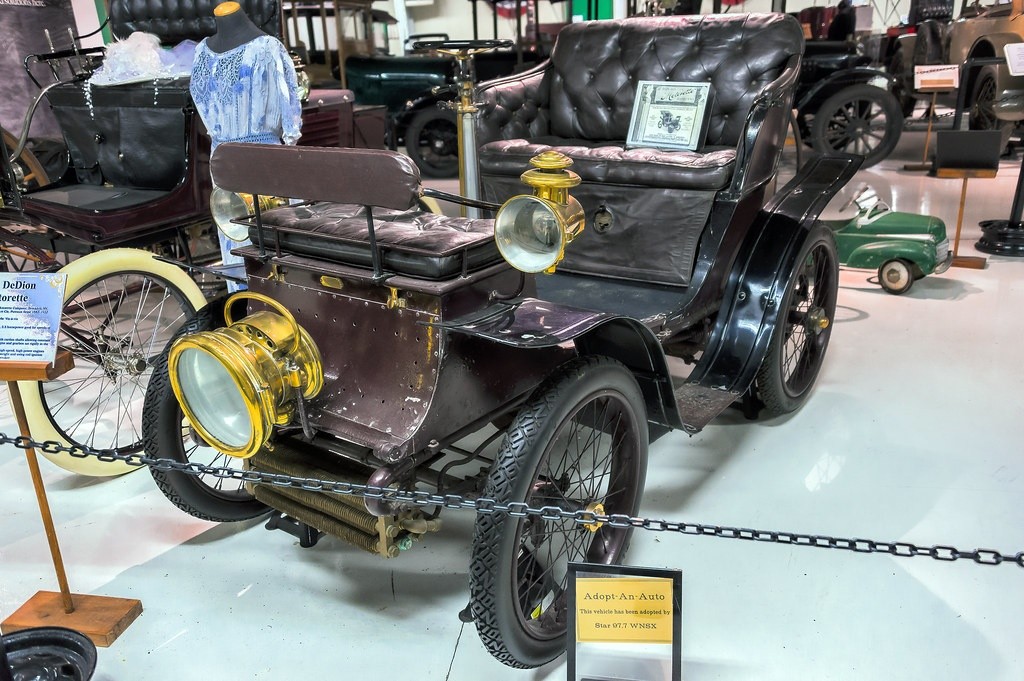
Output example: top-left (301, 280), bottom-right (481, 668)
top-left (187, 2), bottom-right (302, 293)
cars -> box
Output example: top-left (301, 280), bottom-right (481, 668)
top-left (0, 0), bottom-right (1024, 671)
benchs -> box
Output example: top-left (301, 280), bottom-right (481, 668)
top-left (470, 11), bottom-right (806, 201)
top-left (26, 0), bottom-right (289, 110)
top-left (210, 142), bottom-right (515, 297)
